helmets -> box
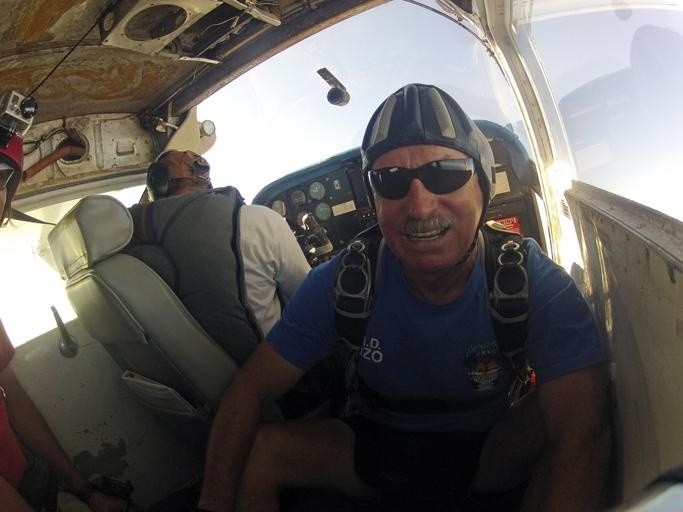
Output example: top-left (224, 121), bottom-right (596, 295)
top-left (361, 83), bottom-right (496, 201)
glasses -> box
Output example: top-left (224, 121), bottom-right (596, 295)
top-left (368, 157), bottom-right (474, 199)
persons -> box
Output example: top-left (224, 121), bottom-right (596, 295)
top-left (147, 150), bottom-right (313, 339)
top-left (0, 135), bottom-right (129, 512)
top-left (196, 83), bottom-right (612, 512)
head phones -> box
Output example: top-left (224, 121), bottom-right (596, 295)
top-left (144, 160), bottom-right (211, 202)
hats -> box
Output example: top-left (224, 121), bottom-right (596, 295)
top-left (151, 151), bottom-right (211, 182)
